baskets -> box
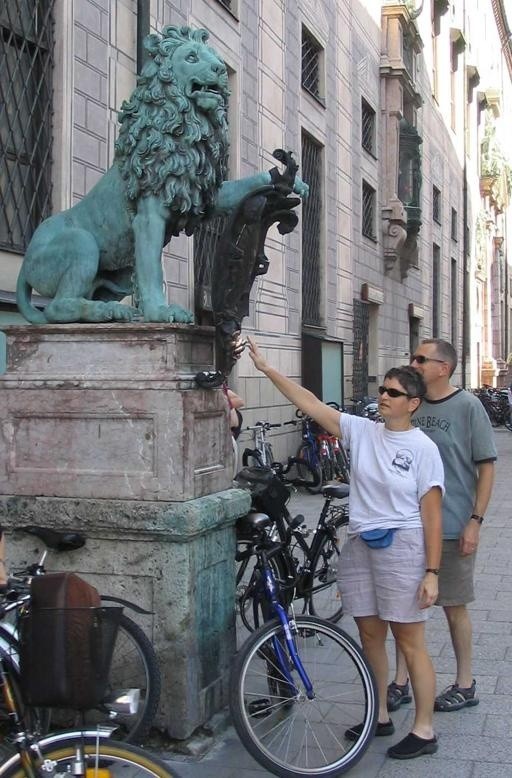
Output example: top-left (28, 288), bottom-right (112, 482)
top-left (22, 605), bottom-right (125, 711)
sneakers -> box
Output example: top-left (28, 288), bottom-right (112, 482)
top-left (346, 718), bottom-right (394, 741)
top-left (388, 731), bottom-right (439, 759)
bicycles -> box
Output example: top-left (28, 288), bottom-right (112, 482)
top-left (0, 561), bottom-right (188, 778)
top-left (473, 382), bottom-right (512, 432)
top-left (232, 510), bottom-right (380, 777)
top-left (284, 395), bottom-right (390, 495)
top-left (242, 420), bottom-right (281, 467)
top-left (0, 523), bottom-right (163, 771)
top-left (235, 448), bottom-right (349, 646)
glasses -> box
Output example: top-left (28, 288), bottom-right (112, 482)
top-left (378, 386), bottom-right (409, 398)
top-left (410, 355), bottom-right (444, 363)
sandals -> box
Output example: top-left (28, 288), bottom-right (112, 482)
top-left (387, 677), bottom-right (413, 710)
top-left (433, 680), bottom-right (479, 711)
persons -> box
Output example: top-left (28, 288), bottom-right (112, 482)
top-left (223, 381), bottom-right (245, 480)
top-left (247, 335), bottom-right (447, 759)
top-left (384, 339), bottom-right (499, 715)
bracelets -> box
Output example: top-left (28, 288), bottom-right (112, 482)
top-left (469, 514), bottom-right (484, 525)
top-left (424, 567), bottom-right (441, 576)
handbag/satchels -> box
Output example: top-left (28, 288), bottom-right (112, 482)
top-left (29, 574), bottom-right (104, 701)
top-left (359, 527), bottom-right (394, 549)
top-left (234, 463), bottom-right (290, 515)
